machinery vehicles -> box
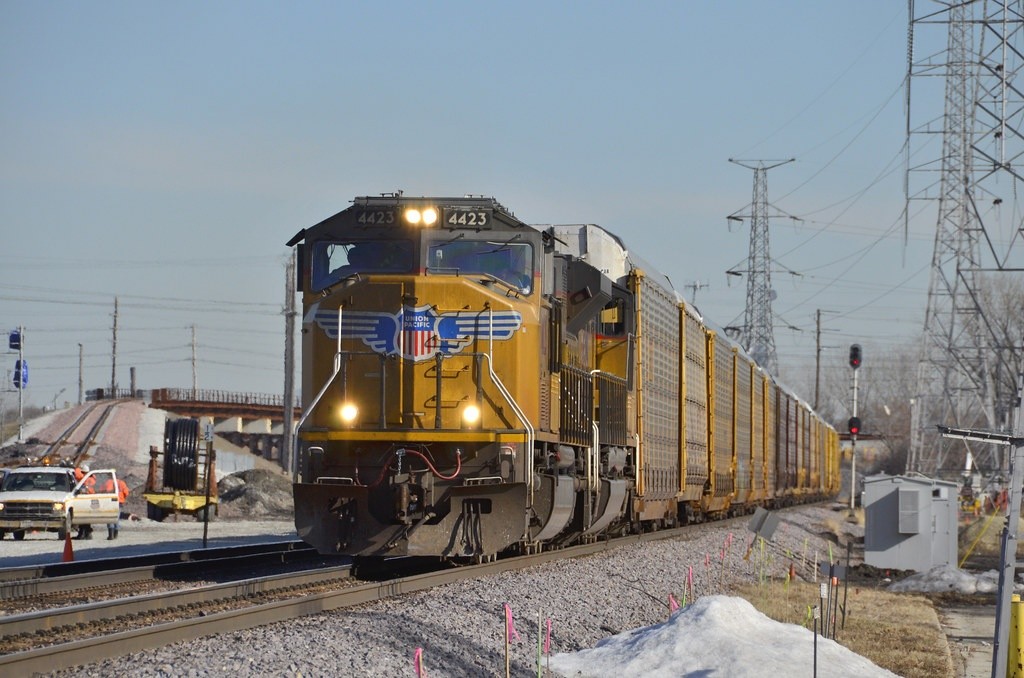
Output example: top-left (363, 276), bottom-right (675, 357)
top-left (140, 419), bottom-right (220, 523)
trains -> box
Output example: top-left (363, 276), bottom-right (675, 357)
top-left (286, 193), bottom-right (840, 566)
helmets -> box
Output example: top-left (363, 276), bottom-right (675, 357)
top-left (80, 464), bottom-right (90, 473)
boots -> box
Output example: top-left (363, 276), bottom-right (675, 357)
top-left (107, 528), bottom-right (118, 540)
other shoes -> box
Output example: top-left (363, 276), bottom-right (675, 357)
top-left (72, 528), bottom-right (93, 540)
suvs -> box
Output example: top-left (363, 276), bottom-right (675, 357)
top-left (0, 465), bottom-right (120, 542)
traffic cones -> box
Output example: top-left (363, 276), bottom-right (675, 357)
top-left (60, 531), bottom-right (75, 561)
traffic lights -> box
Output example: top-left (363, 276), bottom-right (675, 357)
top-left (849, 344), bottom-right (862, 370)
top-left (848, 417), bottom-right (860, 434)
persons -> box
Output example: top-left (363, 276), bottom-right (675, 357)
top-left (99, 468), bottom-right (129, 540)
top-left (71, 464), bottom-right (97, 540)
